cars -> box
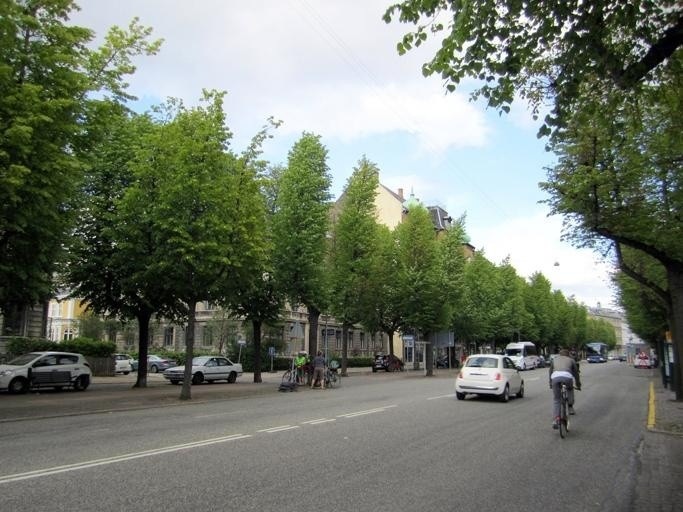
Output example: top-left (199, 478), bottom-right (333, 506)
top-left (455, 354), bottom-right (524, 403)
top-left (608, 353), bottom-right (627, 362)
top-left (437, 355), bottom-right (459, 369)
top-left (165, 355), bottom-right (243, 385)
top-left (633, 354), bottom-right (650, 369)
top-left (538, 354), bottom-right (546, 368)
top-left (131, 355), bottom-right (179, 373)
top-left (0, 351), bottom-right (91, 392)
top-left (113, 353), bottom-right (135, 375)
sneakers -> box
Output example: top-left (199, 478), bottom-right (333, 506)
top-left (568, 406), bottom-right (575, 415)
top-left (552, 416), bottom-right (560, 429)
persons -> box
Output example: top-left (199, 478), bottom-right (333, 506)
top-left (569, 346), bottom-right (577, 361)
top-left (288, 350), bottom-right (340, 389)
top-left (548, 349), bottom-right (581, 429)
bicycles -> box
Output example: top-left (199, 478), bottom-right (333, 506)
top-left (549, 384), bottom-right (581, 439)
top-left (281, 366), bottom-right (341, 390)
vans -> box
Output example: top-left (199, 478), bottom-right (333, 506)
top-left (505, 342), bottom-right (539, 371)
top-left (550, 346), bottom-right (563, 363)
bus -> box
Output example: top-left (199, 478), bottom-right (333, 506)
top-left (585, 341), bottom-right (608, 363)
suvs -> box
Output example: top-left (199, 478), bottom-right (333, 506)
top-left (372, 354), bottom-right (404, 372)
